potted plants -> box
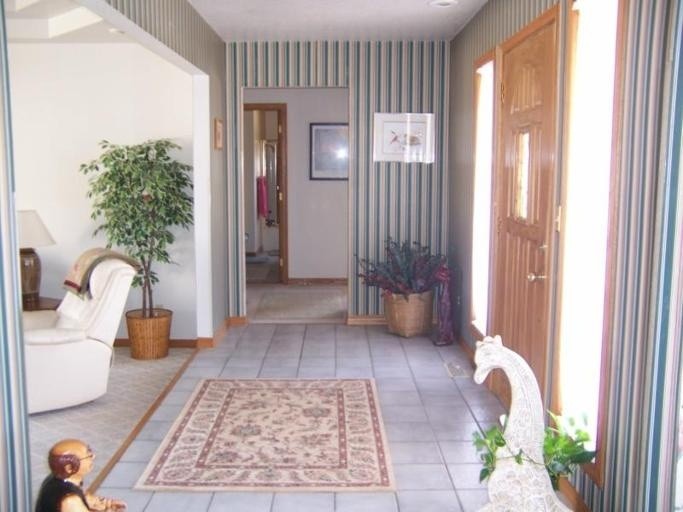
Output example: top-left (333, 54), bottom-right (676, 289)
top-left (352, 231), bottom-right (454, 341)
top-left (78, 132), bottom-right (195, 362)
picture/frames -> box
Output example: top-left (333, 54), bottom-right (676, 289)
top-left (305, 121), bottom-right (349, 182)
top-left (370, 110), bottom-right (436, 167)
top-left (212, 114), bottom-right (223, 152)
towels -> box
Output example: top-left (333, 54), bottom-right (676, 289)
top-left (61, 243), bottom-right (141, 303)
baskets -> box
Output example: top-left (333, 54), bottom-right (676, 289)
top-left (384, 289), bottom-right (434, 338)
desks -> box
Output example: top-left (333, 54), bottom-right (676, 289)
top-left (34, 294), bottom-right (63, 311)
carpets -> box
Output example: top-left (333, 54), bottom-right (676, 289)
top-left (132, 379), bottom-right (394, 493)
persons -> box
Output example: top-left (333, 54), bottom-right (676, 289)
top-left (34, 439), bottom-right (128, 512)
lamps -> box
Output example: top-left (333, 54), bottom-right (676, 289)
top-left (14, 207), bottom-right (58, 311)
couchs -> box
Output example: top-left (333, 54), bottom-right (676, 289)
top-left (14, 252), bottom-right (138, 420)
